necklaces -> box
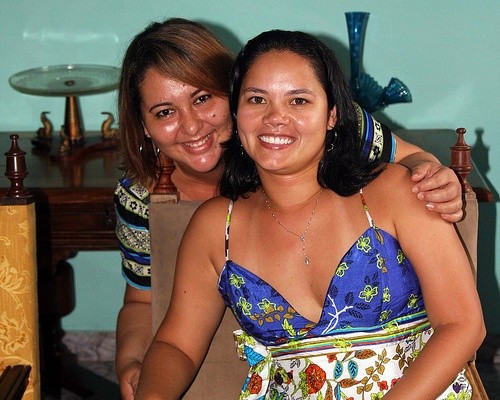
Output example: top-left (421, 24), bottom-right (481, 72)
top-left (260, 187), bottom-right (325, 264)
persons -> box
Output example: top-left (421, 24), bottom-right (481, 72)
top-left (131, 28), bottom-right (488, 400)
top-left (111, 16), bottom-right (466, 400)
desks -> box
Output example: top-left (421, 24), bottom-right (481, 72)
top-left (0, 128), bottom-right (495, 400)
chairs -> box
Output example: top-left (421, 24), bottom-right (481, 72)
top-left (148, 127), bottom-right (488, 399)
